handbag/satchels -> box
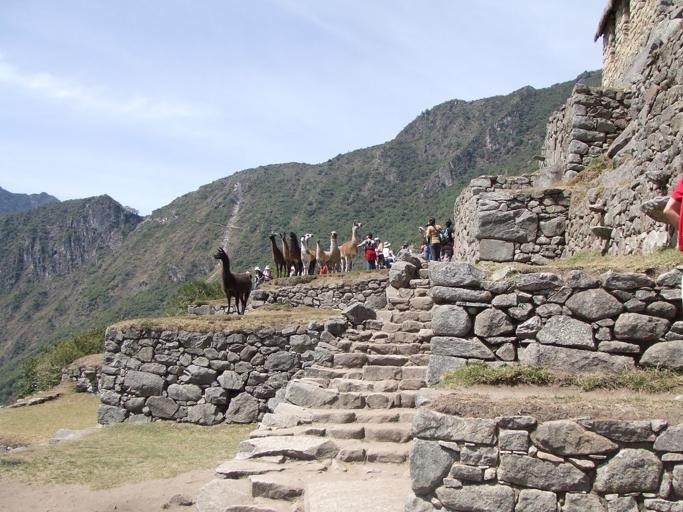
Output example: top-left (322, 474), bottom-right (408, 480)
top-left (441, 236), bottom-right (448, 245)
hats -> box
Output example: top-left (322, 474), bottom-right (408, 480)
top-left (265, 266), bottom-right (271, 270)
top-left (383, 242), bottom-right (391, 247)
top-left (255, 267), bottom-right (260, 270)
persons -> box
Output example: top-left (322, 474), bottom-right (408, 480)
top-left (356, 217), bottom-right (456, 271)
top-left (253, 266), bottom-right (264, 289)
top-left (262, 264), bottom-right (273, 282)
top-left (662, 175), bottom-right (683, 252)
